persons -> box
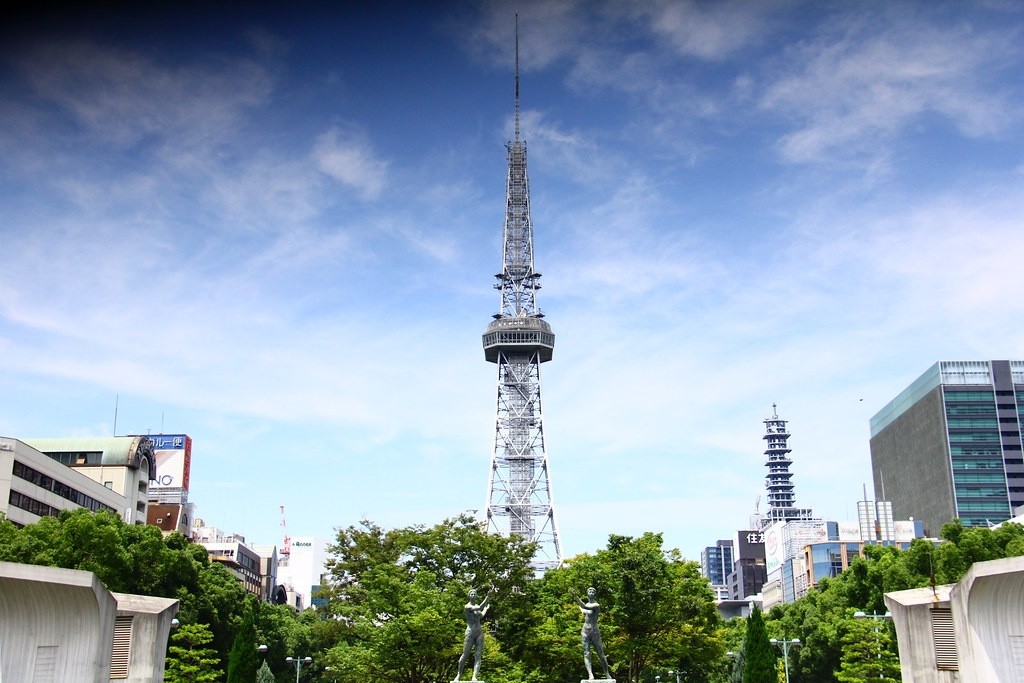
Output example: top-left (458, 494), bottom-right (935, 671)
top-left (569, 587), bottom-right (612, 680)
top-left (452, 584), bottom-right (495, 681)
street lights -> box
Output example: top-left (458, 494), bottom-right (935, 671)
top-left (853, 610), bottom-right (893, 679)
top-left (668, 670), bottom-right (687, 683)
top-left (769, 636), bottom-right (801, 683)
top-left (286, 655), bottom-right (312, 683)
top-left (655, 676), bottom-right (660, 683)
